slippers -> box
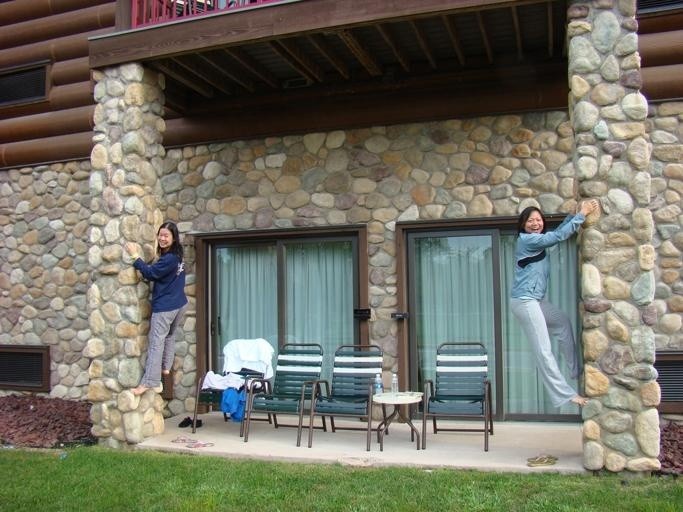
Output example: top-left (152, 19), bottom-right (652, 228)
top-left (527, 454), bottom-right (558, 467)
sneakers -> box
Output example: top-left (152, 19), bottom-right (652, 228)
top-left (178, 417), bottom-right (192, 427)
top-left (192, 419), bottom-right (202, 427)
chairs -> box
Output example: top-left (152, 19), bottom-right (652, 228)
top-left (306, 345), bottom-right (388, 451)
top-left (242, 343), bottom-right (329, 446)
top-left (421, 340), bottom-right (495, 451)
top-left (192, 339), bottom-right (273, 436)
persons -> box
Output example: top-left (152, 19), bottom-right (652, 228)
top-left (123, 221), bottom-right (189, 398)
top-left (508, 198), bottom-right (598, 410)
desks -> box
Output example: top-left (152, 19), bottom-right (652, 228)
top-left (372, 393), bottom-right (423, 451)
